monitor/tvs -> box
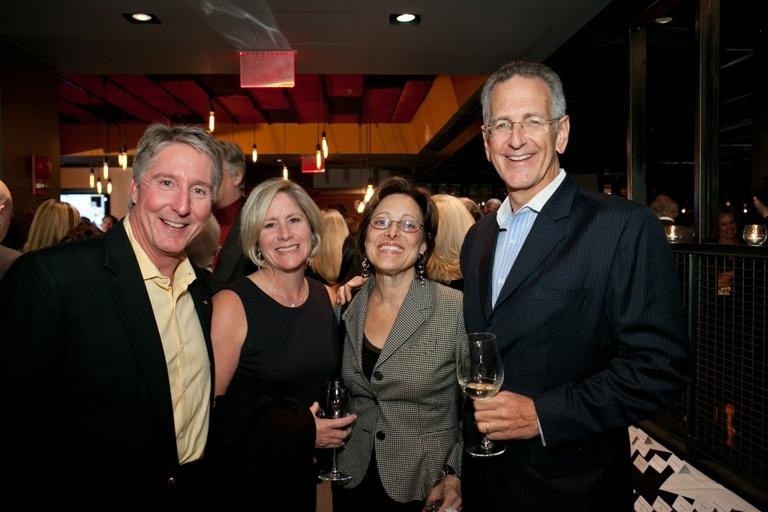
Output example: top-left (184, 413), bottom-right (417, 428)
top-left (61, 188), bottom-right (110, 228)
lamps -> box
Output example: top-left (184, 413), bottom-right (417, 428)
top-left (89, 103), bottom-right (375, 215)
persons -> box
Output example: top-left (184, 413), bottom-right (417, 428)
top-left (328, 172), bottom-right (479, 512)
top-left (206, 176), bottom-right (344, 510)
top-left (649, 190), bottom-right (712, 299)
top-left (707, 207), bottom-right (747, 333)
top-left (338, 58), bottom-right (695, 510)
top-left (0, 138), bottom-right (502, 293)
top-left (740, 179), bottom-right (768, 246)
top-left (0, 120), bottom-right (225, 506)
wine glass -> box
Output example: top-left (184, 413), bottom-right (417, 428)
top-left (317, 378), bottom-right (355, 483)
top-left (454, 330), bottom-right (509, 457)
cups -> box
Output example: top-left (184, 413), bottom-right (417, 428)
top-left (741, 224), bottom-right (767, 245)
top-left (664, 225), bottom-right (685, 244)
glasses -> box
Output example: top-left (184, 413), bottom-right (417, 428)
top-left (367, 216), bottom-right (424, 233)
top-left (484, 117), bottom-right (558, 133)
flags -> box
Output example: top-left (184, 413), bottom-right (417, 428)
top-left (238, 50), bottom-right (297, 91)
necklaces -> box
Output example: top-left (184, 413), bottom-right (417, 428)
top-left (258, 267), bottom-right (309, 310)
